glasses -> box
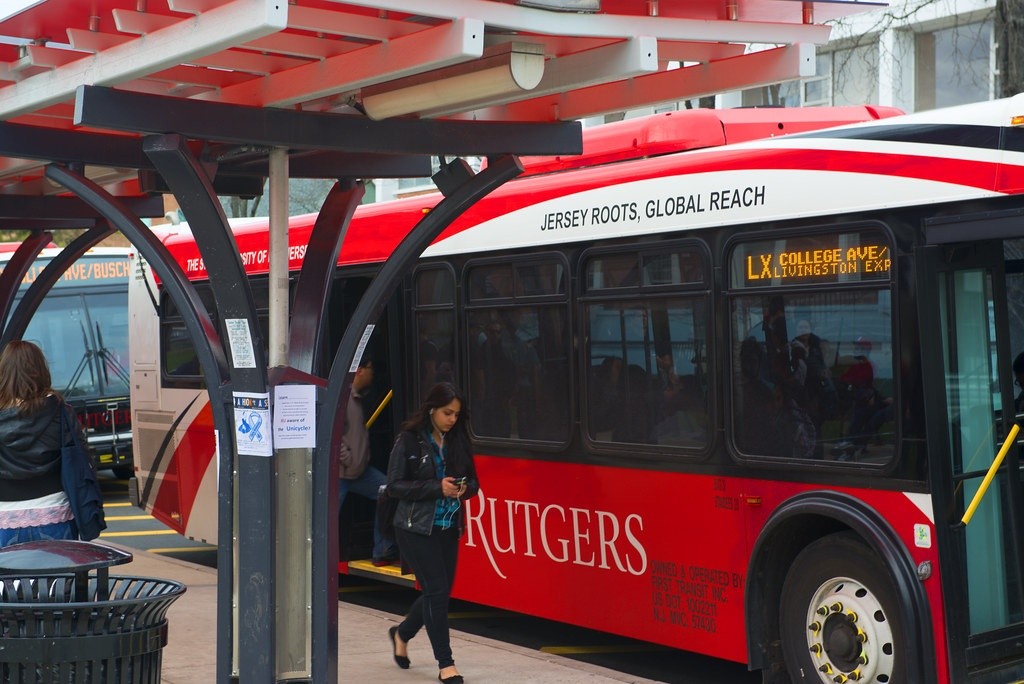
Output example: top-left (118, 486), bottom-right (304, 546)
top-left (1015, 379), bottom-right (1021, 386)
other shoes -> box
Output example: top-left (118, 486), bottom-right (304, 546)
top-left (373, 551), bottom-right (400, 567)
top-left (438, 673), bottom-right (464, 684)
top-left (389, 626), bottom-right (409, 669)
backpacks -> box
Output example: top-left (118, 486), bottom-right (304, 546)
top-left (379, 431), bottom-right (420, 540)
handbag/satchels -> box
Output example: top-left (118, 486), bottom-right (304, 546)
top-left (61, 407), bottom-right (108, 543)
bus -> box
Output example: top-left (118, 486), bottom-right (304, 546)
top-left (125, 88), bottom-right (1024, 684)
top-left (0, 239), bottom-right (135, 483)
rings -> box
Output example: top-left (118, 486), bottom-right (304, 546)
top-left (449, 492), bottom-right (451, 494)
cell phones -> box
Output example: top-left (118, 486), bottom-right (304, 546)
top-left (452, 476), bottom-right (467, 485)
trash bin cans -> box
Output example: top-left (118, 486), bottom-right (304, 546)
top-left (0, 538), bottom-right (189, 684)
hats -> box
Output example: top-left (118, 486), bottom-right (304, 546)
top-left (852, 336), bottom-right (871, 352)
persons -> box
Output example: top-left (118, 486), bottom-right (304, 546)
top-left (103, 346), bottom-right (129, 377)
top-left (0, 340), bottom-right (109, 598)
top-left (419, 326), bottom-right (437, 381)
top-left (596, 352), bottom-right (630, 410)
top-left (338, 351), bottom-right (400, 568)
top-left (386, 383), bottom-right (478, 684)
top-left (1013, 351), bottom-right (1024, 411)
top-left (469, 306), bottom-right (542, 387)
top-left (731, 311), bottom-right (893, 460)
